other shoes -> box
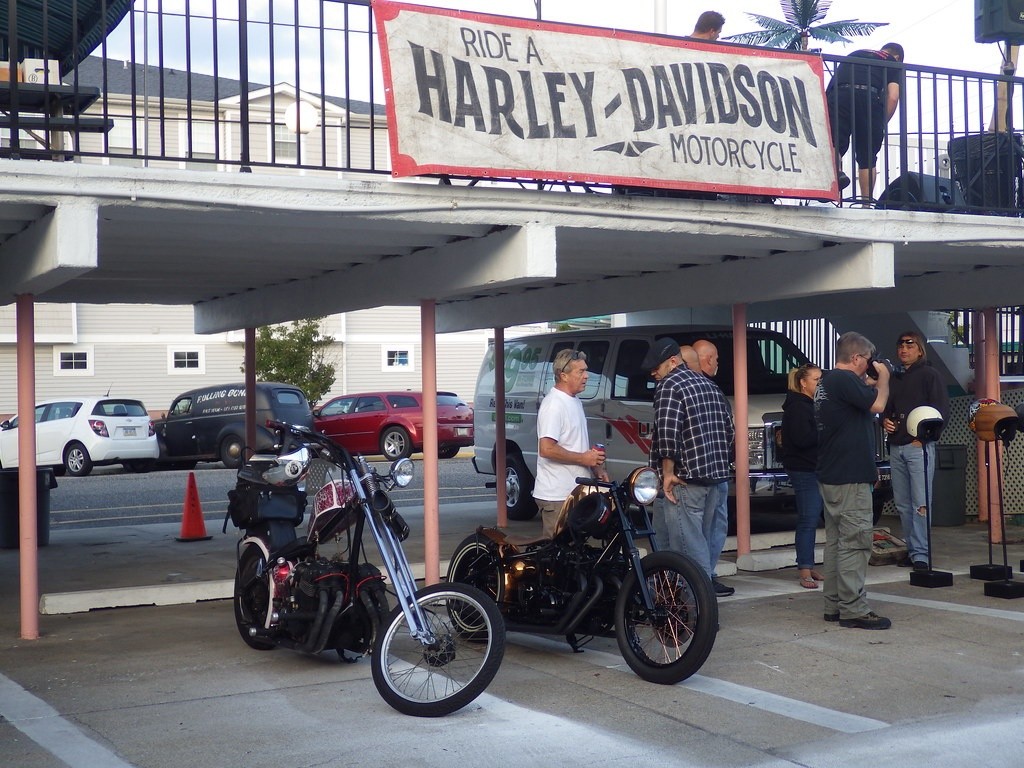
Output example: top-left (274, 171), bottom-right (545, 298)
top-left (913, 560), bottom-right (927, 571)
top-left (897, 556), bottom-right (914, 567)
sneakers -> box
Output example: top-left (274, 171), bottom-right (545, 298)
top-left (838, 611), bottom-right (891, 629)
top-left (824, 613), bottom-right (839, 621)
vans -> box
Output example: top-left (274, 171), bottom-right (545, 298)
top-left (473, 325), bottom-right (890, 526)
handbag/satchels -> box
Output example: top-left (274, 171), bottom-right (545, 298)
top-left (227, 485), bottom-right (307, 528)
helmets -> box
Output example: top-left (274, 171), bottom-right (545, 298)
top-left (906, 407), bottom-right (943, 440)
top-left (968, 398), bottom-right (1002, 432)
top-left (976, 406), bottom-right (1019, 442)
top-left (1014, 401), bottom-right (1024, 434)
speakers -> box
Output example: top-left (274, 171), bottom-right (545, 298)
top-left (974, 0), bottom-right (1024, 46)
top-left (874, 170), bottom-right (966, 215)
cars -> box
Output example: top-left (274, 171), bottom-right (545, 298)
top-left (0, 395), bottom-right (160, 476)
top-left (313, 391), bottom-right (474, 460)
top-left (129, 381), bottom-right (316, 472)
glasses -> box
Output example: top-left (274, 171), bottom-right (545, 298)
top-left (897, 338), bottom-right (915, 345)
top-left (562, 348), bottom-right (584, 372)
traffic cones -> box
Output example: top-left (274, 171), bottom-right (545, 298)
top-left (173, 473), bottom-right (214, 541)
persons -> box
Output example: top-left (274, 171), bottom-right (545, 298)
top-left (814, 332), bottom-right (891, 630)
top-left (532, 350), bottom-right (609, 539)
top-left (879, 332), bottom-right (951, 571)
top-left (689, 10), bottom-right (725, 41)
top-left (826, 42), bottom-right (904, 207)
top-left (642, 338), bottom-right (735, 596)
top-left (781, 363), bottom-right (822, 589)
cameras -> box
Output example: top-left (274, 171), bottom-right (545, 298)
top-left (866, 352), bottom-right (906, 381)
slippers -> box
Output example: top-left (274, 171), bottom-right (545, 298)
top-left (800, 577), bottom-right (819, 588)
top-left (810, 570), bottom-right (828, 582)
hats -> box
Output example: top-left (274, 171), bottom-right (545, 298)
top-left (641, 338), bottom-right (681, 370)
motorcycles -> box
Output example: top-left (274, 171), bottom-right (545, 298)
top-left (224, 419), bottom-right (509, 718)
top-left (447, 476), bottom-right (718, 685)
